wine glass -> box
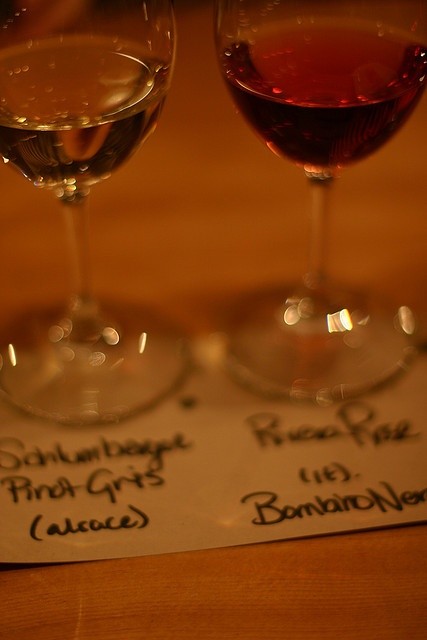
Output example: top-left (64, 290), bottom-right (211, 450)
top-left (214, 0), bottom-right (427, 399)
top-left (0, 0), bottom-right (190, 425)
